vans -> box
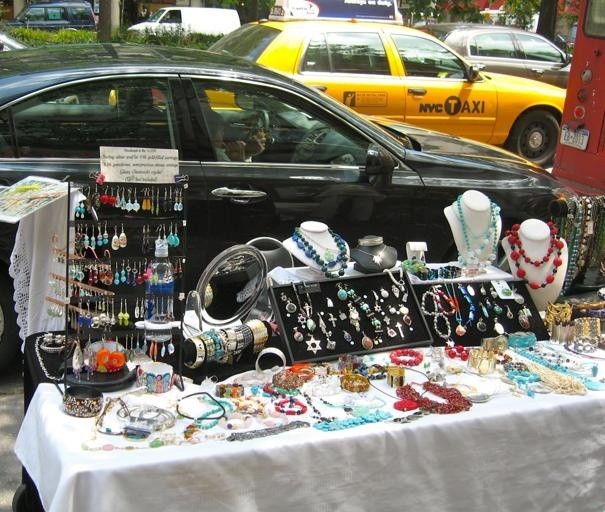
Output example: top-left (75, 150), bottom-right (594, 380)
top-left (126, 6), bottom-right (241, 39)
top-left (476, 8), bottom-right (540, 36)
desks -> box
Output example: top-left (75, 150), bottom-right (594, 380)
top-left (12, 337), bottom-right (604, 512)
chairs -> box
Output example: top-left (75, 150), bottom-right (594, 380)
top-left (246, 237), bottom-right (294, 276)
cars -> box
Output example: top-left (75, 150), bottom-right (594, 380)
top-left (414, 24), bottom-right (571, 89)
top-left (0, 33), bottom-right (29, 52)
top-left (107, 1), bottom-right (569, 165)
top-left (0, 40), bottom-right (569, 380)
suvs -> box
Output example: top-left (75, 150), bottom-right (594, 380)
top-left (5, 2), bottom-right (96, 30)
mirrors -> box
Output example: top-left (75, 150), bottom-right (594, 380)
top-left (183, 243), bottom-right (268, 333)
